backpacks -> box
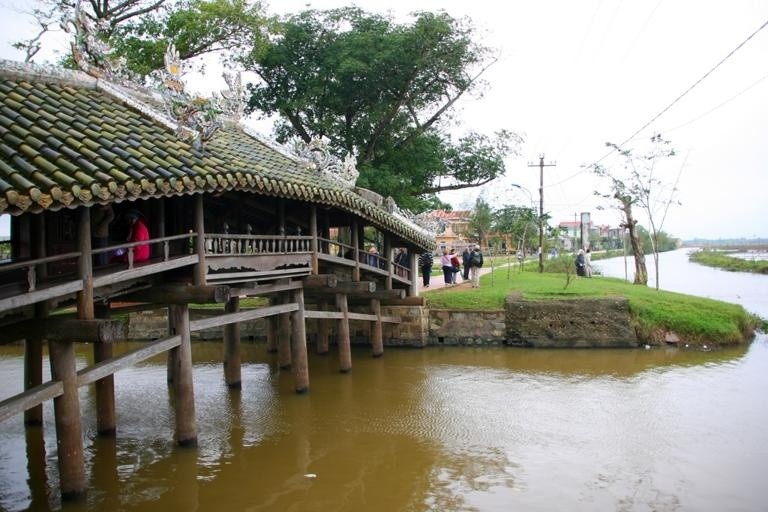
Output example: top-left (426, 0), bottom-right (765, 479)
top-left (470, 251), bottom-right (480, 264)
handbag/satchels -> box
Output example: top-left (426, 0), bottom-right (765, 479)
top-left (423, 255), bottom-right (431, 267)
top-left (452, 266), bottom-right (458, 272)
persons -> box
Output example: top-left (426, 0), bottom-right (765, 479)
top-left (551, 248), bottom-right (557, 256)
top-left (90, 203), bottom-right (115, 268)
top-left (395, 248), bottom-right (409, 280)
top-left (441, 244), bottom-right (483, 288)
top-left (573, 248), bottom-right (594, 278)
top-left (110, 209), bottom-right (150, 263)
top-left (365, 243), bottom-right (378, 267)
top-left (419, 251), bottom-right (433, 288)
top-left (517, 248), bottom-right (524, 263)
